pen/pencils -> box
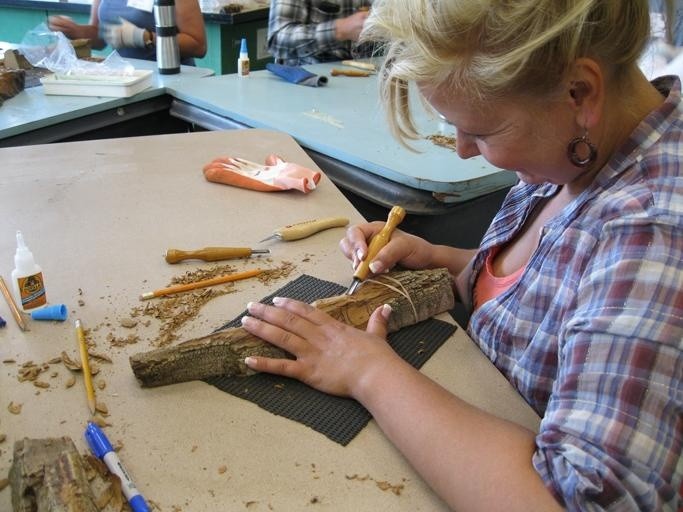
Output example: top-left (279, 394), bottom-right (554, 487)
top-left (75, 318), bottom-right (96, 415)
top-left (0, 277), bottom-right (25, 330)
top-left (86, 421), bottom-right (149, 512)
top-left (139, 269), bottom-right (270, 300)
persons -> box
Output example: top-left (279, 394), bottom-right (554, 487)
top-left (266, 0), bottom-right (406, 70)
top-left (239, 0), bottom-right (681, 512)
top-left (45, 0), bottom-right (208, 68)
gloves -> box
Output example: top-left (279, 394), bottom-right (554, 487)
top-left (204, 155), bottom-right (320, 194)
top-left (266, 63), bottom-right (327, 87)
top-left (102, 18), bottom-right (145, 48)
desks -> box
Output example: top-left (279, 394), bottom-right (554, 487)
top-left (0, 129), bottom-right (538, 512)
top-left (1, 42), bottom-right (215, 147)
top-left (166, 40), bottom-right (539, 249)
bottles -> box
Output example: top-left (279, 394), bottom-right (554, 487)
top-left (153, 0), bottom-right (181, 75)
